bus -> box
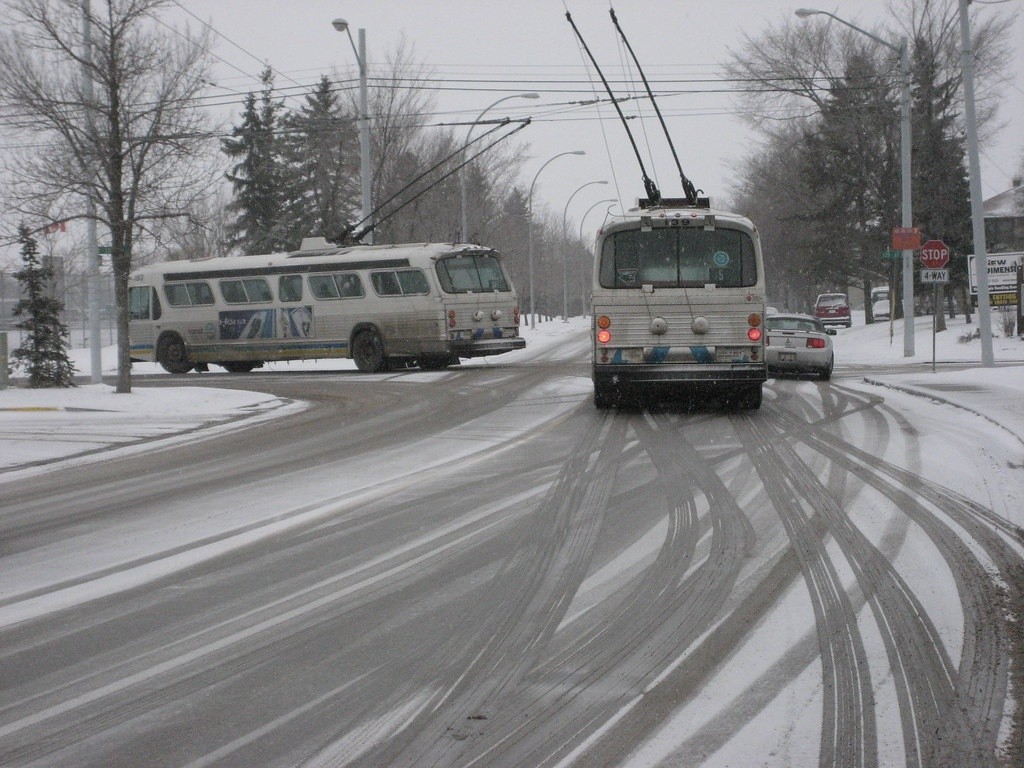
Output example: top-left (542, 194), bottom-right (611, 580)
top-left (565, 10), bottom-right (766, 410)
top-left (121, 121), bottom-right (528, 374)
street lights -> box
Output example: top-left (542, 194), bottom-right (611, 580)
top-left (458, 92), bottom-right (540, 245)
top-left (564, 180), bottom-right (618, 246)
top-left (793, 5), bottom-right (915, 358)
top-left (527, 150), bottom-right (586, 328)
top-left (332, 16), bottom-right (372, 244)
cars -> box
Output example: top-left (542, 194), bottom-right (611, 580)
top-left (813, 293), bottom-right (852, 328)
top-left (765, 307), bottom-right (837, 380)
top-left (870, 286), bottom-right (890, 318)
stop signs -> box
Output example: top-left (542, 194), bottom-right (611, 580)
top-left (918, 239), bottom-right (950, 268)
